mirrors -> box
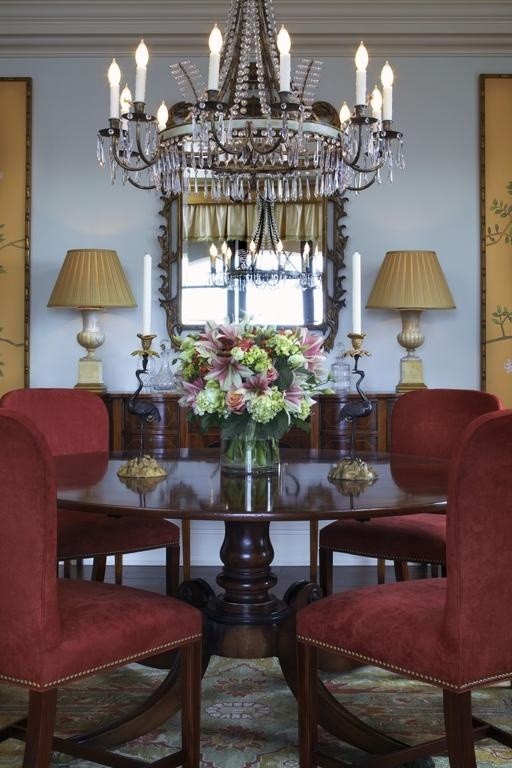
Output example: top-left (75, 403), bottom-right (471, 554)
top-left (153, 182), bottom-right (348, 357)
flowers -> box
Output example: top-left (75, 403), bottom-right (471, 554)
top-left (169, 325), bottom-right (332, 462)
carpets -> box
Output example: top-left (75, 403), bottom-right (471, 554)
top-left (1, 632), bottom-right (511, 767)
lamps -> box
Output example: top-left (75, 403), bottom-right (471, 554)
top-left (98, 2), bottom-right (410, 286)
top-left (46, 249), bottom-right (139, 384)
top-left (368, 250), bottom-right (459, 393)
top-left (202, 197), bottom-right (323, 289)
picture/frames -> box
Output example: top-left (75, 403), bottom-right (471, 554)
top-left (477, 70), bottom-right (512, 406)
top-left (1, 73), bottom-right (31, 393)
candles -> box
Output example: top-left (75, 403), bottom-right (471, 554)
top-left (349, 250), bottom-right (363, 334)
top-left (140, 251), bottom-right (152, 336)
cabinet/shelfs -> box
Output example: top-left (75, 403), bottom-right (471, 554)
top-left (104, 392), bottom-right (185, 455)
top-left (317, 395), bottom-right (394, 456)
top-left (185, 395), bottom-right (316, 451)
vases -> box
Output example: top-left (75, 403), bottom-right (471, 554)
top-left (214, 389), bottom-right (284, 480)
top-left (219, 471), bottom-right (280, 515)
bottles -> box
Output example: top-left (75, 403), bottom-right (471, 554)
top-left (329, 343), bottom-right (351, 398)
top-left (155, 340), bottom-right (177, 393)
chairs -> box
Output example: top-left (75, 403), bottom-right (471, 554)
top-left (314, 386), bottom-right (504, 607)
top-left (1, 413), bottom-right (202, 766)
top-left (296, 407), bottom-right (511, 766)
top-left (2, 387), bottom-right (182, 596)
